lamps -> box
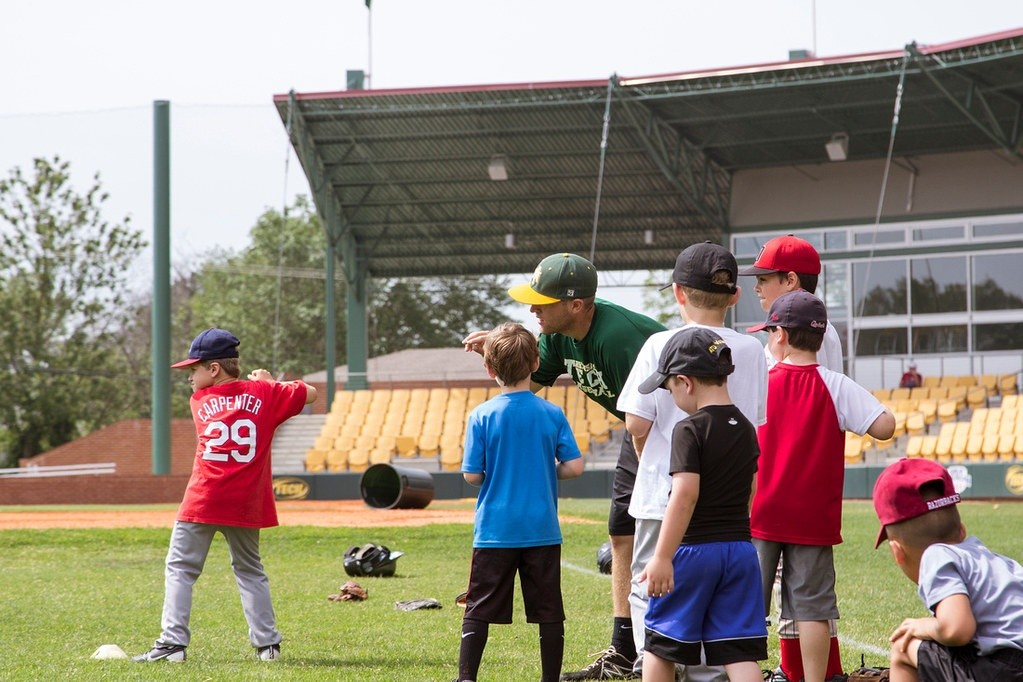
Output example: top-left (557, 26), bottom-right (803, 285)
top-left (488, 154), bottom-right (511, 182)
top-left (824, 132), bottom-right (851, 161)
top-left (505, 232), bottom-right (519, 246)
top-left (644, 229), bottom-right (652, 244)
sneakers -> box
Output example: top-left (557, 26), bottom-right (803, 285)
top-left (560, 646), bottom-right (641, 682)
top-left (256, 643), bottom-right (280, 662)
top-left (762, 665), bottom-right (805, 682)
top-left (133, 646), bottom-right (187, 662)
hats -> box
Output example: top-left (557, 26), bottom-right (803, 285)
top-left (507, 253), bottom-right (598, 305)
top-left (171, 328), bottom-right (240, 368)
top-left (873, 458), bottom-right (961, 549)
top-left (745, 288), bottom-right (828, 334)
top-left (658, 241), bottom-right (738, 295)
top-left (738, 234), bottom-right (821, 276)
top-left (637, 327), bottom-right (735, 394)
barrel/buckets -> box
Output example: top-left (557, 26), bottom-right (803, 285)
top-left (359, 462), bottom-right (434, 509)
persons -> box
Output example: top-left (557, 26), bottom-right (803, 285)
top-left (899, 363), bottom-right (923, 388)
top-left (636, 328), bottom-right (769, 682)
top-left (131, 328), bottom-right (319, 663)
top-left (873, 457), bottom-right (1023, 682)
top-left (462, 253), bottom-right (669, 682)
top-left (616, 240), bottom-right (768, 682)
top-left (454, 322), bottom-right (584, 682)
top-left (738, 234), bottom-right (897, 682)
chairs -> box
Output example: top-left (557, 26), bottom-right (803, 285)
top-left (841, 373), bottom-right (1023, 467)
top-left (301, 380), bottom-right (627, 473)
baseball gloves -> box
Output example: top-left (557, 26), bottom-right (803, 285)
top-left (847, 653), bottom-right (890, 682)
top-left (455, 592), bottom-right (467, 608)
top-left (328, 581), bottom-right (369, 602)
top-left (394, 597), bottom-right (444, 613)
